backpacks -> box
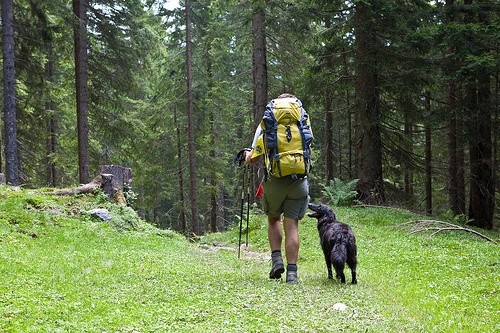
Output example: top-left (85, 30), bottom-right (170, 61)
top-left (261, 98), bottom-right (315, 180)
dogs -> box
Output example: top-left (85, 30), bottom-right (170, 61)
top-left (305, 203), bottom-right (358, 285)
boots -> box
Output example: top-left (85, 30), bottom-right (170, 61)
top-left (268, 252), bottom-right (285, 279)
top-left (286, 265), bottom-right (298, 284)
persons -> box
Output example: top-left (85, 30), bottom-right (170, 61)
top-left (244, 93), bottom-right (314, 285)
